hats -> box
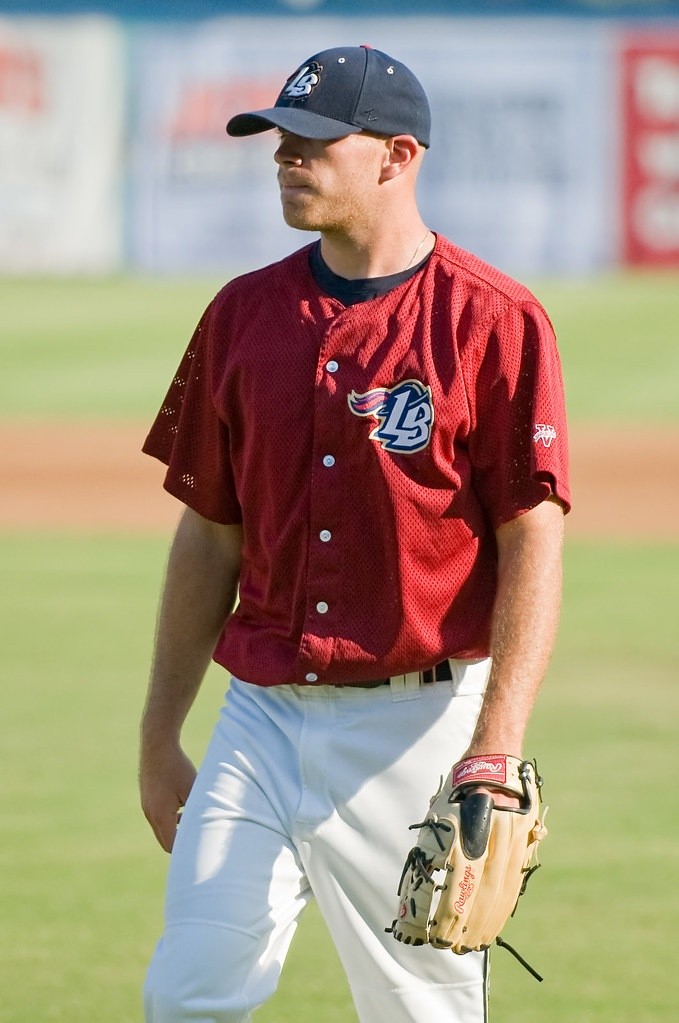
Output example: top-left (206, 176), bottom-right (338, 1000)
top-left (226, 44), bottom-right (431, 149)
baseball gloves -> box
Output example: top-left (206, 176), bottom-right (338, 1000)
top-left (383, 752), bottom-right (550, 982)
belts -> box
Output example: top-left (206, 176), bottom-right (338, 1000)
top-left (329, 659), bottom-right (452, 688)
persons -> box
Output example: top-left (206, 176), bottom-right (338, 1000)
top-left (138, 44), bottom-right (573, 1022)
